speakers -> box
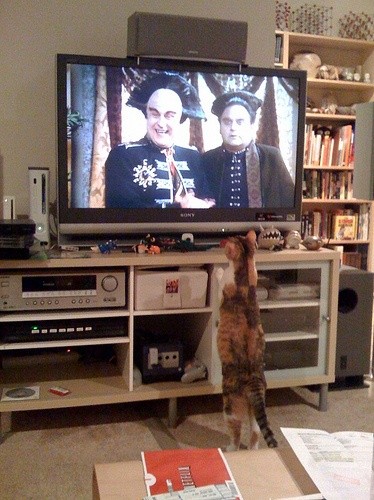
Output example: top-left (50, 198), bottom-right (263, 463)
top-left (335, 268), bottom-right (374, 388)
top-left (28, 166), bottom-right (51, 253)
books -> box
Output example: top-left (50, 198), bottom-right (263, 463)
top-left (331, 203), bottom-right (369, 240)
top-left (301, 208), bottom-right (332, 240)
top-left (324, 244), bottom-right (368, 271)
top-left (304, 122), bottom-right (356, 167)
top-left (302, 169), bottom-right (354, 201)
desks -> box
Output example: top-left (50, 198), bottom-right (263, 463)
top-left (93, 427), bottom-right (374, 500)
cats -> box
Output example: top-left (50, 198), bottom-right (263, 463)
top-left (212, 226), bottom-right (278, 452)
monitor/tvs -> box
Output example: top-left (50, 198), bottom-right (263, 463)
top-left (57, 53), bottom-right (308, 246)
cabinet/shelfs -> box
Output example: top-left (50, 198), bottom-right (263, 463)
top-left (217, 243), bottom-right (341, 413)
top-left (0, 250), bottom-right (130, 433)
top-left (275, 28), bottom-right (374, 274)
top-left (131, 250), bottom-right (217, 426)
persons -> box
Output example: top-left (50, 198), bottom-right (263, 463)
top-left (105, 67), bottom-right (216, 209)
top-left (204, 91), bottom-right (295, 209)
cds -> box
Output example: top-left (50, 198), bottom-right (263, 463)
top-left (5, 388), bottom-right (36, 398)
